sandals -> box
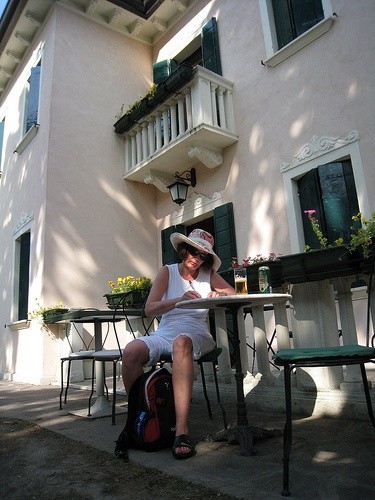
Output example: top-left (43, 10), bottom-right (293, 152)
top-left (172, 434), bottom-right (195, 460)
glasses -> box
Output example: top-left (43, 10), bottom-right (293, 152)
top-left (189, 248), bottom-right (209, 262)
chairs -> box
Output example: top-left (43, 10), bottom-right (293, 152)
top-left (61, 271), bottom-right (375, 496)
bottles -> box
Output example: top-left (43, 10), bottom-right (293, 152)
top-left (136, 411), bottom-right (146, 436)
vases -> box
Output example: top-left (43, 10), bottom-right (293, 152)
top-left (276, 240), bottom-right (375, 286)
top-left (43, 308), bottom-right (68, 324)
top-left (103, 289), bottom-right (150, 309)
top-left (218, 261), bottom-right (279, 290)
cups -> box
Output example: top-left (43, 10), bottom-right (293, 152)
top-left (234, 268), bottom-right (248, 294)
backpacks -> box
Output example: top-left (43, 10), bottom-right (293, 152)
top-left (113, 365), bottom-right (175, 464)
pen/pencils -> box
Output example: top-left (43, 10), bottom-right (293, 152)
top-left (189, 280), bottom-right (197, 292)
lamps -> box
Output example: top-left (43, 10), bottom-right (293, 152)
top-left (166, 166), bottom-right (197, 206)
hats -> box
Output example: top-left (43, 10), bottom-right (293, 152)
top-left (170, 229), bottom-right (223, 273)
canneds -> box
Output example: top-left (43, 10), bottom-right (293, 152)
top-left (258, 265), bottom-right (272, 293)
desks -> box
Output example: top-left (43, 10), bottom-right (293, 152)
top-left (43, 310), bottom-right (142, 420)
top-left (174, 292), bottom-right (293, 456)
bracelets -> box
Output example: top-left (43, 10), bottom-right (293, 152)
top-left (218, 291), bottom-right (227, 296)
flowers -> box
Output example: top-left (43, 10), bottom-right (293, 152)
top-left (107, 275), bottom-right (153, 295)
top-left (304, 206), bottom-right (375, 250)
top-left (26, 299), bottom-right (69, 320)
top-left (231, 252), bottom-right (278, 268)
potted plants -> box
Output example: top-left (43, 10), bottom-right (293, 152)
top-left (113, 62), bottom-right (194, 134)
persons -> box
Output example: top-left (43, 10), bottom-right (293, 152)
top-left (122, 230), bottom-right (237, 460)
